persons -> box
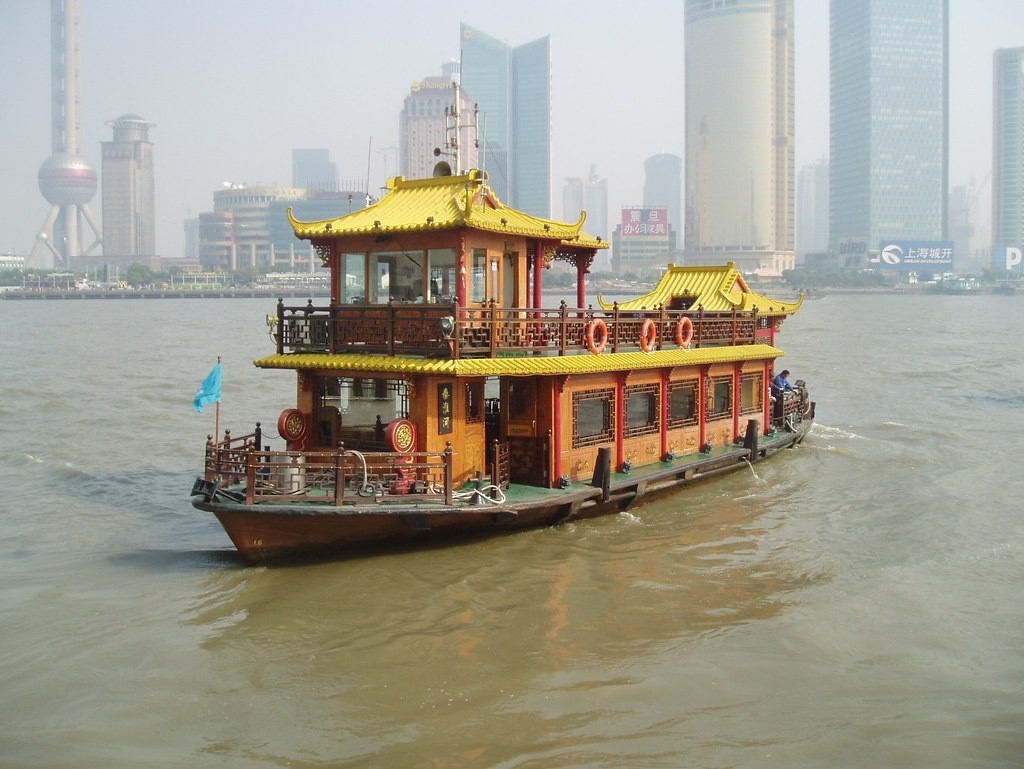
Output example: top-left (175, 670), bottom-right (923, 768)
top-left (412, 269), bottom-right (438, 302)
top-left (769, 370), bottom-right (798, 427)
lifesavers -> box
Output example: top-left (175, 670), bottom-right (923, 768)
top-left (585, 316), bottom-right (609, 353)
top-left (640, 320), bottom-right (658, 352)
top-left (676, 317), bottom-right (694, 347)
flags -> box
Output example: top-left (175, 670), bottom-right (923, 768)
top-left (193, 362), bottom-right (221, 412)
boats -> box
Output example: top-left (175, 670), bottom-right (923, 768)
top-left (190, 79), bottom-right (815, 566)
top-left (762, 286), bottom-right (826, 301)
